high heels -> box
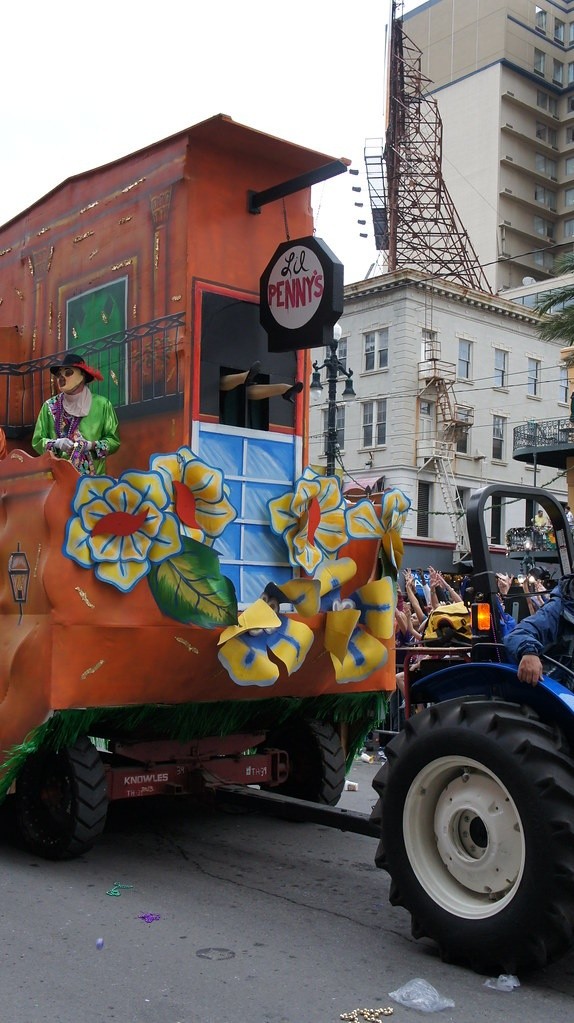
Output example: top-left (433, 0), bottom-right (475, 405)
top-left (282, 381), bottom-right (303, 404)
top-left (244, 361), bottom-right (262, 388)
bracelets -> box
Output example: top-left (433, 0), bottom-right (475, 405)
top-left (525, 652), bottom-right (538, 656)
top-left (430, 591), bottom-right (436, 593)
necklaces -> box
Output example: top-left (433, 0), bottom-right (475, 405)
top-left (69, 440), bottom-right (88, 470)
top-left (54, 393), bottom-right (82, 438)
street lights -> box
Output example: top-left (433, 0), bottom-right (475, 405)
top-left (310, 323), bottom-right (356, 477)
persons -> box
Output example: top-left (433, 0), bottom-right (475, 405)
top-left (363, 566), bottom-right (554, 751)
top-left (221, 361), bottom-right (304, 403)
top-left (31, 354), bottom-right (121, 475)
top-left (505, 573), bottom-right (574, 693)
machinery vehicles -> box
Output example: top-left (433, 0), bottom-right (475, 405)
top-left (367, 483), bottom-right (574, 985)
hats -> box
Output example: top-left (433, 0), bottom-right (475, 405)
top-left (49, 353), bottom-right (101, 384)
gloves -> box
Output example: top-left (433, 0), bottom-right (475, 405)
top-left (54, 438), bottom-right (74, 452)
top-left (74, 436), bottom-right (92, 453)
